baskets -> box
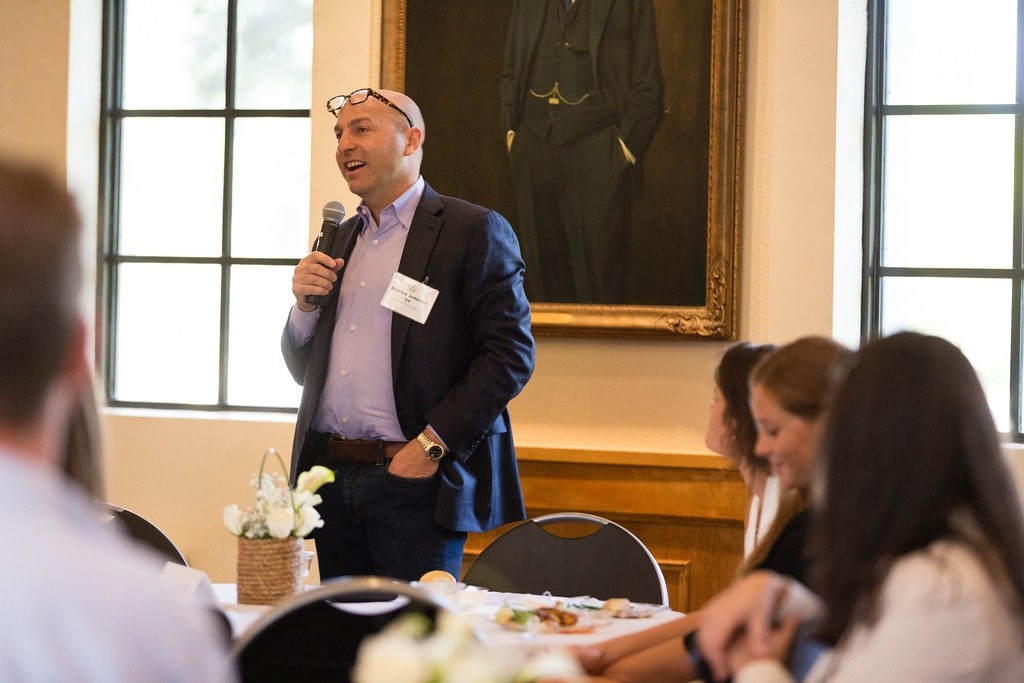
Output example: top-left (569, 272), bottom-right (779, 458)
top-left (238, 449), bottom-right (306, 605)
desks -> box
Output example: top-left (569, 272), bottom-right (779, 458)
top-left (213, 584), bottom-right (703, 683)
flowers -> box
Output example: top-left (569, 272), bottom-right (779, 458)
top-left (220, 461), bottom-right (335, 541)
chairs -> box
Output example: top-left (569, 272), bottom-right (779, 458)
top-left (460, 512), bottom-right (670, 607)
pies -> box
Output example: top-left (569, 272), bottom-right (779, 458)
top-left (495, 604), bottom-right (612, 633)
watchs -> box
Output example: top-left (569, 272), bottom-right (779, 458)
top-left (417, 434), bottom-right (446, 461)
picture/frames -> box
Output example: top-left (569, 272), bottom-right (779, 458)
top-left (376, 0), bottom-right (746, 342)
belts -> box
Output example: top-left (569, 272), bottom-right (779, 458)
top-left (308, 432), bottom-right (409, 464)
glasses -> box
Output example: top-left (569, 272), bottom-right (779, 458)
top-left (327, 88), bottom-right (413, 129)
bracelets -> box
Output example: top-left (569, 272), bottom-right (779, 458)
top-left (685, 630), bottom-right (713, 683)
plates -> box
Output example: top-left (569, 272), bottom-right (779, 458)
top-left (495, 606), bottom-right (613, 637)
top-left (568, 598), bottom-right (673, 624)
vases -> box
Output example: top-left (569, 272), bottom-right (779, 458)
top-left (235, 539), bottom-right (303, 605)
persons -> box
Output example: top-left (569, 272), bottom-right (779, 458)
top-left (0, 156), bottom-right (239, 683)
top-left (280, 88), bottom-right (536, 589)
top-left (589, 332), bottom-right (1024, 683)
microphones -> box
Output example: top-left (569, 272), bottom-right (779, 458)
top-left (305, 201), bottom-right (345, 305)
